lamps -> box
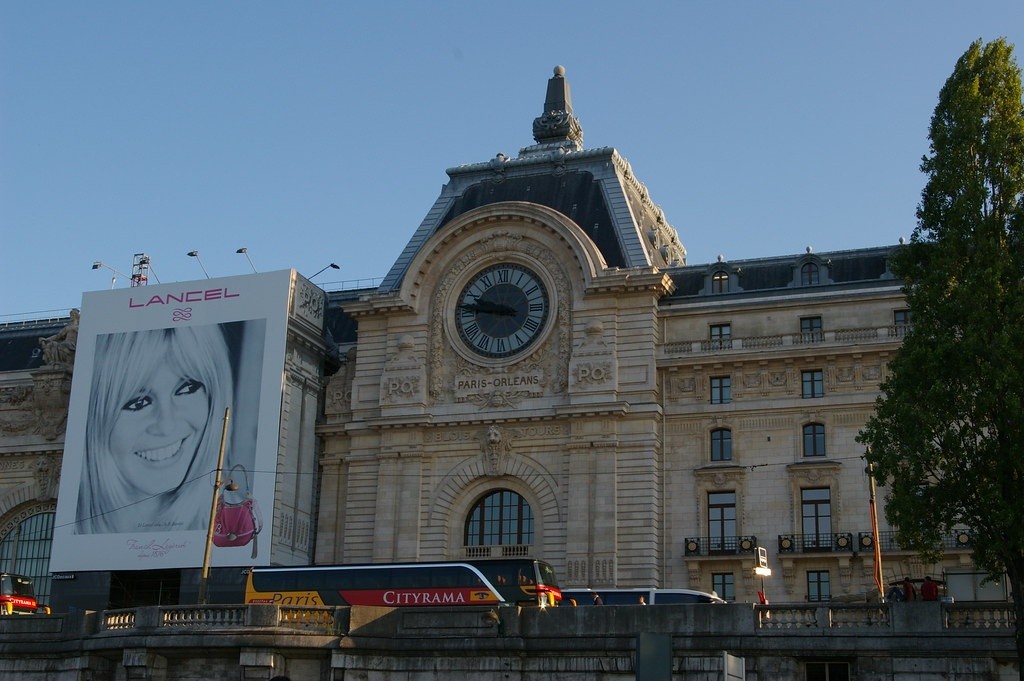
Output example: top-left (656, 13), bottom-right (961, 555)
top-left (235, 247), bottom-right (257, 274)
top-left (306, 263), bottom-right (341, 280)
top-left (91, 261), bottom-right (143, 286)
top-left (187, 250), bottom-right (210, 279)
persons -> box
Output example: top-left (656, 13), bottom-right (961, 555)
top-left (72, 323), bottom-right (234, 535)
top-left (637, 595), bottom-right (648, 606)
top-left (887, 576), bottom-right (916, 602)
top-left (920, 576), bottom-right (938, 601)
top-left (591, 591), bottom-right (603, 605)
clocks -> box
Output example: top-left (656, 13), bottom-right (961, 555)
top-left (442, 252), bottom-right (558, 368)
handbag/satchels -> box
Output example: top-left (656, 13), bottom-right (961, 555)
top-left (210, 464), bottom-right (263, 559)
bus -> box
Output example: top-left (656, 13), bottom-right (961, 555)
top-left (1, 571), bottom-right (50, 623)
top-left (244, 559), bottom-right (577, 632)
top-left (556, 582), bottom-right (729, 605)
